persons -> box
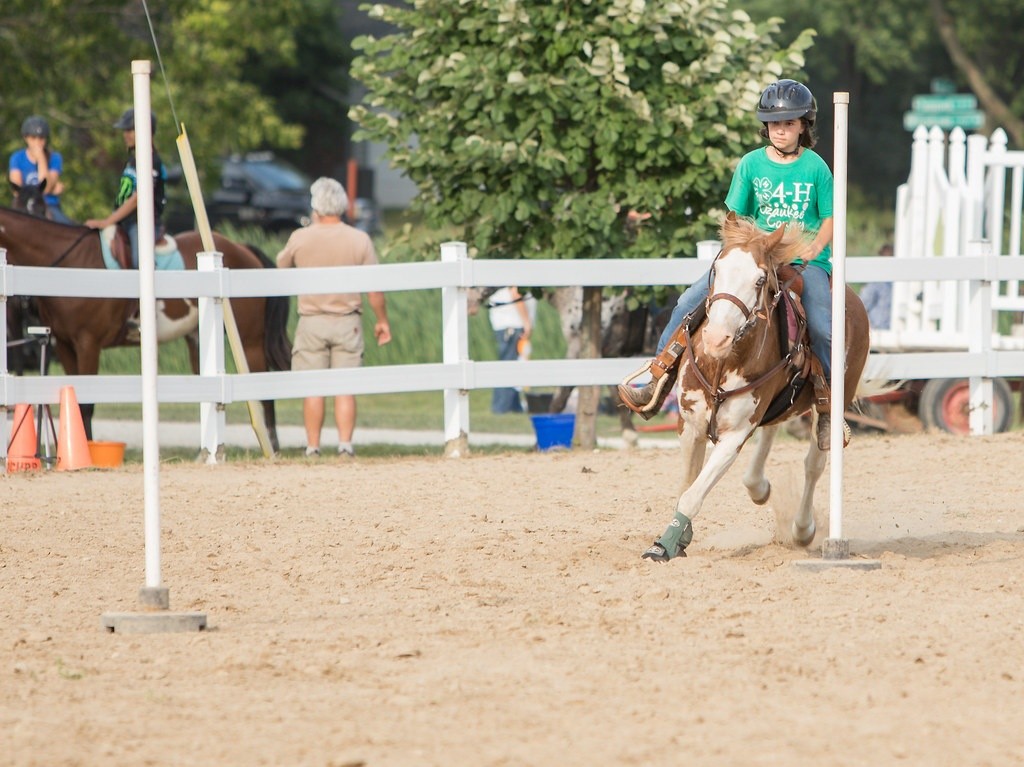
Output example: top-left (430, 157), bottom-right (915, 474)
top-left (85, 106), bottom-right (167, 330)
top-left (468, 286), bottom-right (536, 412)
top-left (616, 79), bottom-right (853, 453)
top-left (273, 176), bottom-right (393, 456)
top-left (9, 114), bottom-right (70, 227)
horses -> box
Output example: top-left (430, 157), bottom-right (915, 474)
top-left (644, 210), bottom-right (869, 561)
top-left (2, 206), bottom-right (295, 457)
top-left (468, 287), bottom-right (673, 452)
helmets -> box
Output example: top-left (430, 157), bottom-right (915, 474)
top-left (21, 115), bottom-right (50, 137)
top-left (112, 107), bottom-right (158, 130)
top-left (755, 79), bottom-right (812, 121)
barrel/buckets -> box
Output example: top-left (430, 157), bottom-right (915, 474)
top-left (88, 441), bottom-right (125, 468)
top-left (529, 414), bottom-right (575, 450)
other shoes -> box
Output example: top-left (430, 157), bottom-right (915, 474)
top-left (304, 450), bottom-right (321, 460)
top-left (816, 412), bottom-right (831, 452)
top-left (337, 448), bottom-right (354, 460)
top-left (617, 383), bottom-right (667, 422)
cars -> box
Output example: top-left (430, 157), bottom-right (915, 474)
top-left (158, 156), bottom-right (382, 240)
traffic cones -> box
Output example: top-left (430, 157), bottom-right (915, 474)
top-left (6, 404), bottom-right (42, 477)
top-left (46, 383), bottom-right (94, 474)
